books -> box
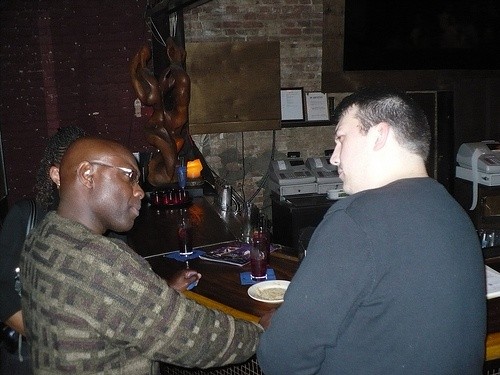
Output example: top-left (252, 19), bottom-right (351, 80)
top-left (198, 243), bottom-right (278, 267)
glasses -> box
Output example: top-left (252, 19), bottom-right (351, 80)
top-left (75, 160), bottom-right (139, 184)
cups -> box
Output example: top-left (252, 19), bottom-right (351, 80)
top-left (177, 223), bottom-right (193, 256)
top-left (150, 187), bottom-right (189, 207)
top-left (249, 237), bottom-right (270, 279)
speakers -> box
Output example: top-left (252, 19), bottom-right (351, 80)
top-left (404, 90), bottom-right (455, 197)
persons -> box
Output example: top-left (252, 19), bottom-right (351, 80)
top-left (257, 89), bottom-right (487, 375)
top-left (0, 125), bottom-right (90, 375)
top-left (19, 137), bottom-right (275, 375)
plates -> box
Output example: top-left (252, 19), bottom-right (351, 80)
top-left (248, 279), bottom-right (291, 303)
